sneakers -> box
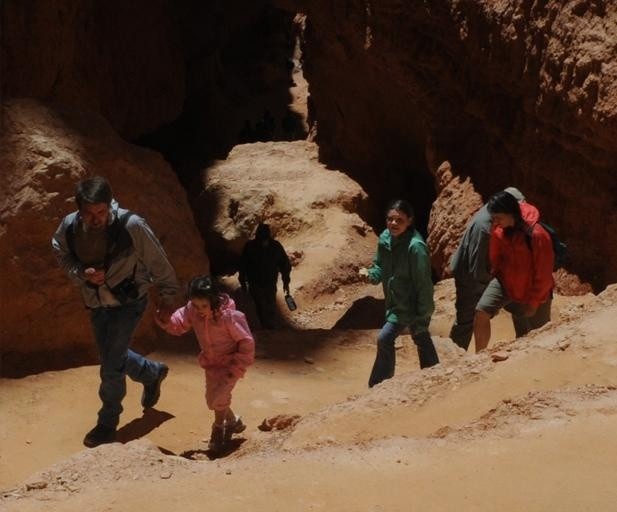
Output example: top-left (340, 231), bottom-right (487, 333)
top-left (225, 418), bottom-right (245, 441)
top-left (141, 362), bottom-right (168, 407)
top-left (208, 423), bottom-right (224, 451)
top-left (84, 423), bottom-right (116, 447)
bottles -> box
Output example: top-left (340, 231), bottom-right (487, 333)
top-left (285, 295), bottom-right (297, 312)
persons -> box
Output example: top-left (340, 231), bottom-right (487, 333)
top-left (357, 198), bottom-right (438, 388)
top-left (448, 186), bottom-right (529, 352)
top-left (52, 177), bottom-right (181, 446)
top-left (154, 275), bottom-right (256, 452)
top-left (239, 224), bottom-right (291, 332)
top-left (473, 190), bottom-right (555, 352)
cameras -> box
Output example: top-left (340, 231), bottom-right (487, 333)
top-left (110, 276), bottom-right (140, 304)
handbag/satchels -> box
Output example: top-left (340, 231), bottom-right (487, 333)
top-left (526, 221), bottom-right (566, 265)
